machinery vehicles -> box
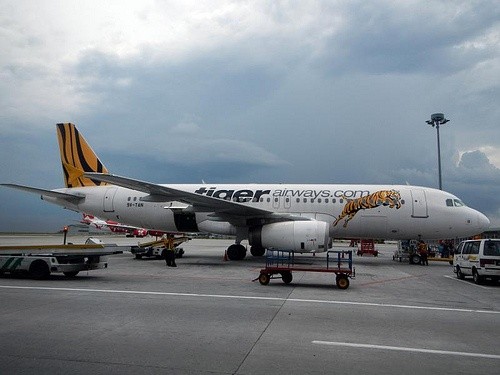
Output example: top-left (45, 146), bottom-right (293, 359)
top-left (1, 233), bottom-right (193, 281)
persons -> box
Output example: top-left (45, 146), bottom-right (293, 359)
top-left (418, 240), bottom-right (429, 265)
top-left (161, 235), bottom-right (177, 268)
top-left (428, 242), bottom-right (457, 262)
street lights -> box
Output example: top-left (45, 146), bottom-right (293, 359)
top-left (426, 113), bottom-right (451, 191)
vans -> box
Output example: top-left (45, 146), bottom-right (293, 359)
top-left (452, 239), bottom-right (500, 283)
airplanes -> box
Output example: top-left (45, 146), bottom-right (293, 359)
top-left (0, 123), bottom-right (492, 259)
